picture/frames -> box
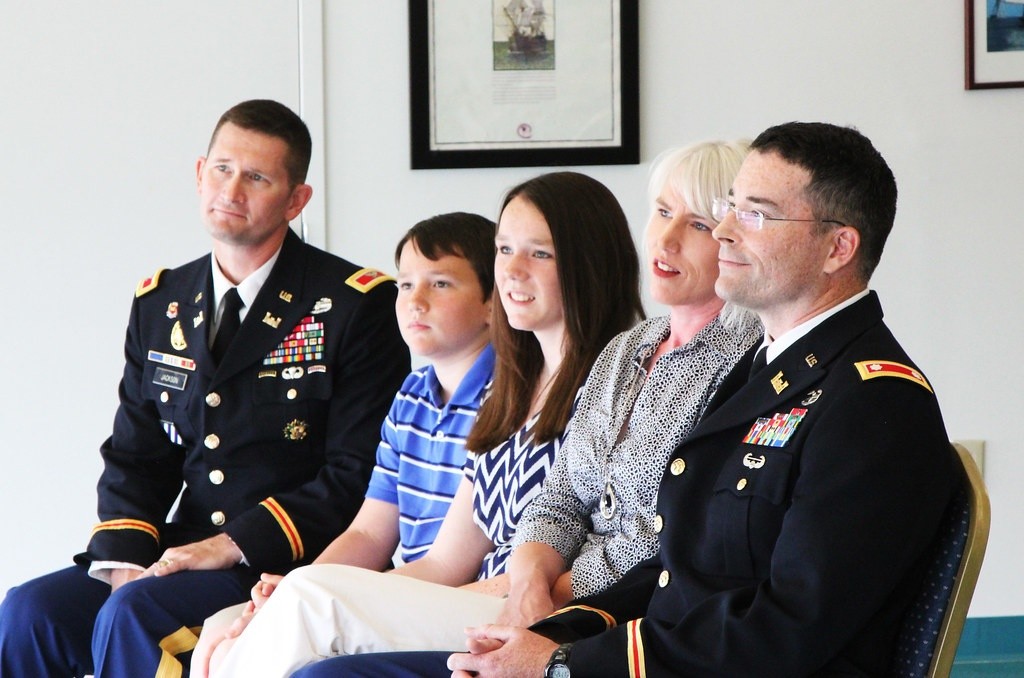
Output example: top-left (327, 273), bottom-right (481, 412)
top-left (964, 0), bottom-right (1024, 92)
top-left (409, 1), bottom-right (640, 171)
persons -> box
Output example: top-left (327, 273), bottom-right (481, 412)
top-left (209, 139), bottom-right (765, 678)
top-left (1, 99), bottom-right (413, 678)
top-left (190, 173), bottom-right (648, 678)
top-left (243, 212), bottom-right (496, 609)
top-left (288, 122), bottom-right (949, 677)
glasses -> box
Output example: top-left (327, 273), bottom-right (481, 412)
top-left (711, 197), bottom-right (847, 232)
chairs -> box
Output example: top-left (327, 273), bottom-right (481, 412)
top-left (885, 443), bottom-right (992, 678)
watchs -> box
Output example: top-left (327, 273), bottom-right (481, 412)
top-left (544, 644), bottom-right (571, 678)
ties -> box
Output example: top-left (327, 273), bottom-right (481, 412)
top-left (210, 288), bottom-right (245, 371)
top-left (749, 346), bottom-right (768, 381)
